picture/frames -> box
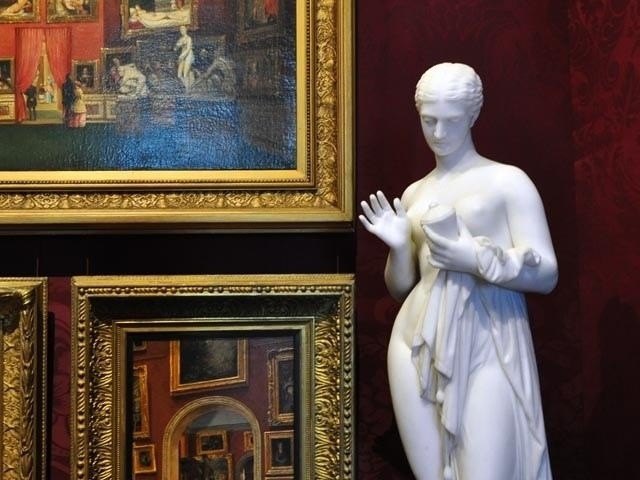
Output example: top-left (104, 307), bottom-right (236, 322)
top-left (1, 1), bottom-right (355, 238)
top-left (1, 274), bottom-right (52, 479)
top-left (68, 272), bottom-right (354, 475)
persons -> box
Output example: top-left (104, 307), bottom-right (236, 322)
top-left (358, 62), bottom-right (559, 480)
top-left (24, 84), bottom-right (37, 120)
top-left (173, 26), bottom-right (193, 93)
top-left (108, 58), bottom-right (150, 95)
top-left (62, 73), bottom-right (86, 128)
